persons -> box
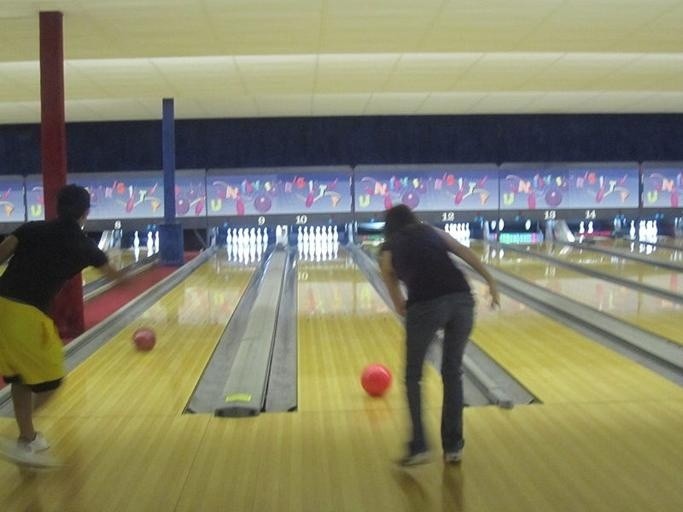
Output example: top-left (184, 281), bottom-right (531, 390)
top-left (0, 183), bottom-right (126, 471)
top-left (377, 203), bottom-right (501, 467)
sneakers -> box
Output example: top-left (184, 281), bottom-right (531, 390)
top-left (399, 449), bottom-right (428, 467)
top-left (17, 431), bottom-right (48, 457)
top-left (443, 447), bottom-right (465, 463)
